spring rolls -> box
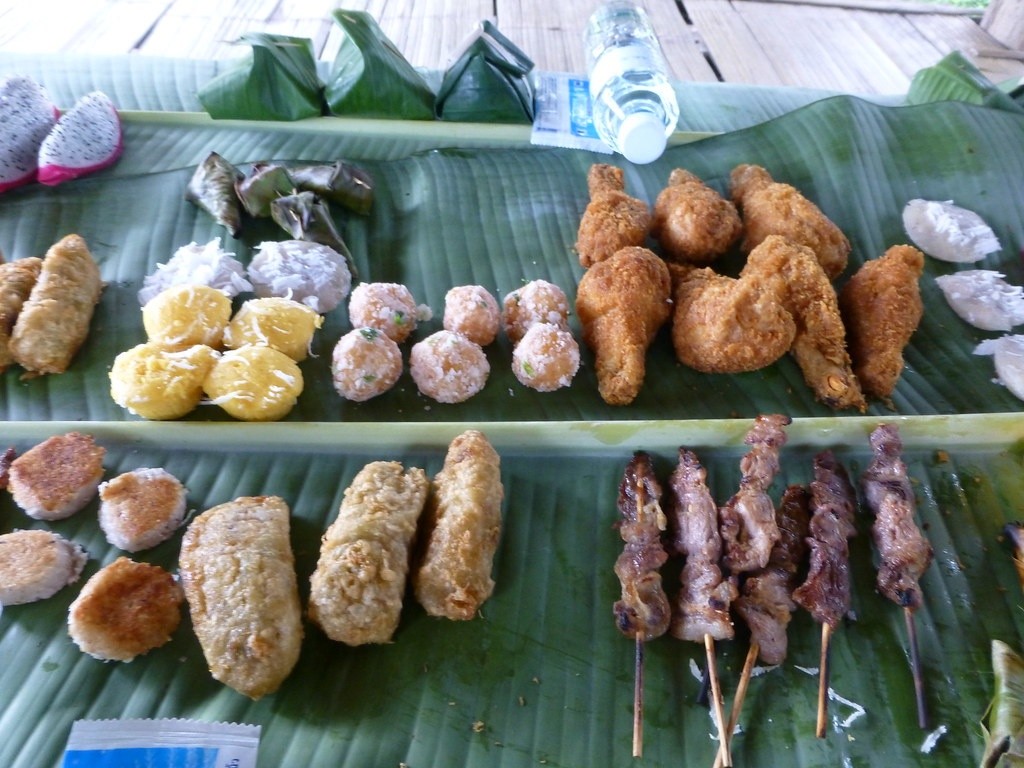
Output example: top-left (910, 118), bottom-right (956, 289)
top-left (303, 429), bottom-right (502, 645)
top-left (0, 233), bottom-right (106, 379)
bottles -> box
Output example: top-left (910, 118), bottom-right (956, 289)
top-left (581, 0), bottom-right (681, 164)
